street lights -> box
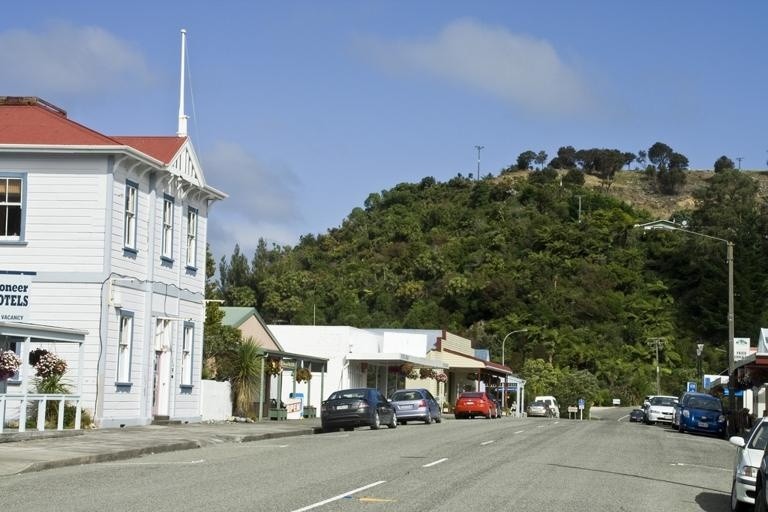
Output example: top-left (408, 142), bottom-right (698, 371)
top-left (644, 336), bottom-right (668, 397)
top-left (695, 348), bottom-right (700, 392)
top-left (695, 343), bottom-right (704, 393)
top-left (663, 225), bottom-right (737, 438)
top-left (474, 144), bottom-right (485, 183)
top-left (502, 329), bottom-right (528, 408)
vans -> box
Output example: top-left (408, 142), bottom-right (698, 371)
top-left (672, 390), bottom-right (732, 438)
top-left (535, 395), bottom-right (561, 418)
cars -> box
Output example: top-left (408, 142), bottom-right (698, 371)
top-left (527, 401), bottom-right (553, 418)
top-left (729, 415), bottom-right (768, 512)
top-left (321, 388), bottom-right (397, 431)
top-left (754, 441), bottom-right (768, 512)
top-left (629, 393), bottom-right (679, 426)
top-left (454, 391), bottom-right (497, 419)
top-left (389, 388), bottom-right (441, 425)
top-left (470, 394), bottom-right (502, 418)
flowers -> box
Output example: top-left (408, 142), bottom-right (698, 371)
top-left (399, 362), bottom-right (448, 384)
